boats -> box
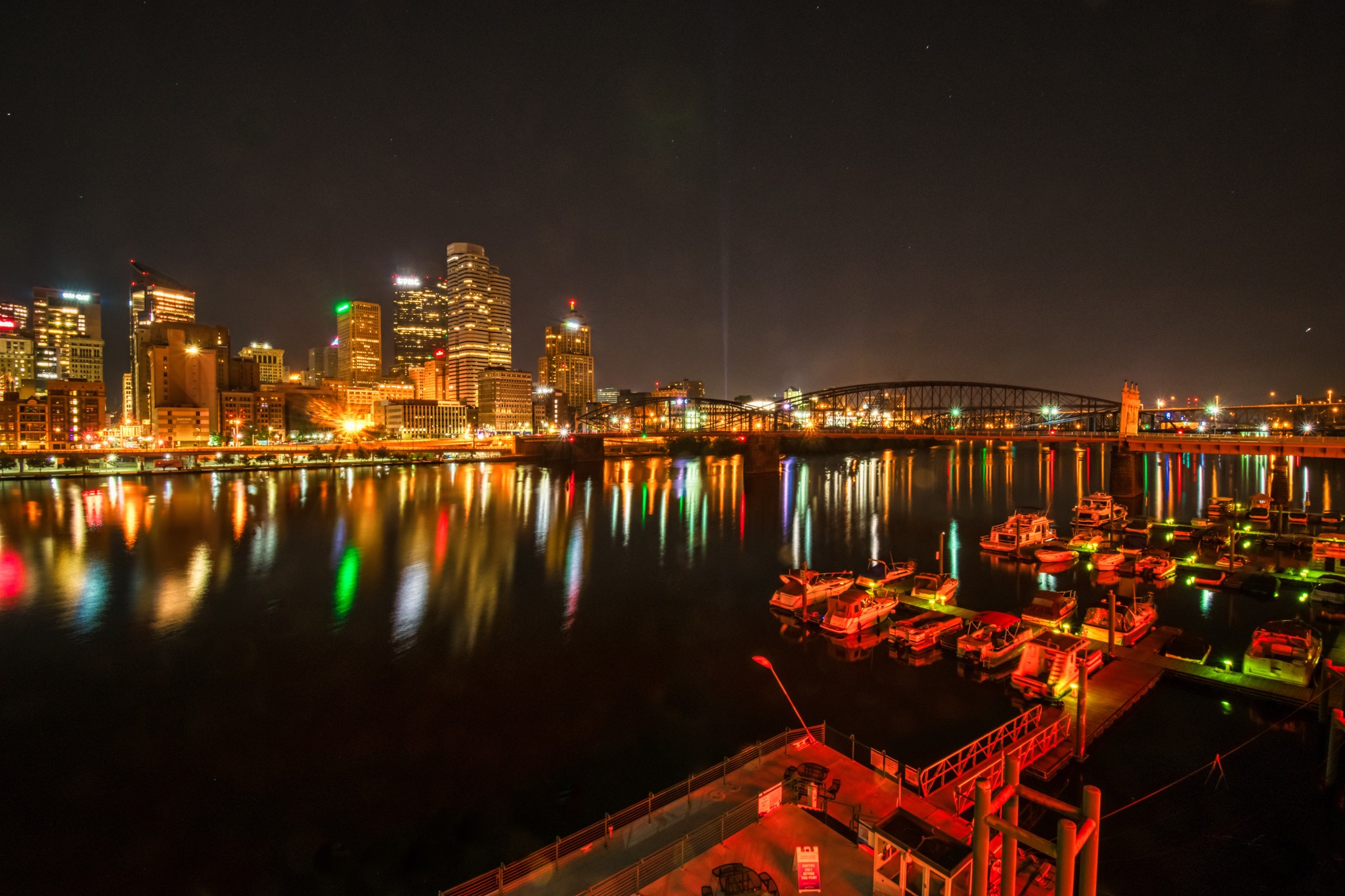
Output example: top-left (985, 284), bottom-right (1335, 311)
top-left (769, 492), bottom-right (1345, 708)
